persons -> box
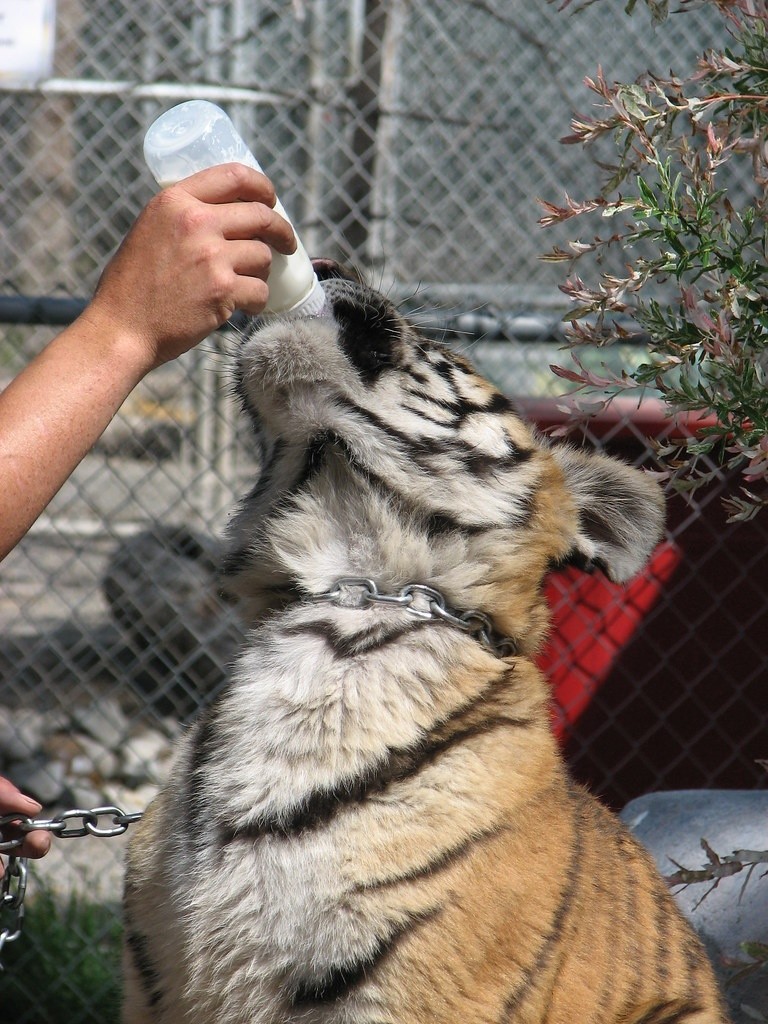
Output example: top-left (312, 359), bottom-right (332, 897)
top-left (0, 162), bottom-right (297, 879)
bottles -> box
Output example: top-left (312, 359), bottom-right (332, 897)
top-left (142, 99), bottom-right (325, 322)
